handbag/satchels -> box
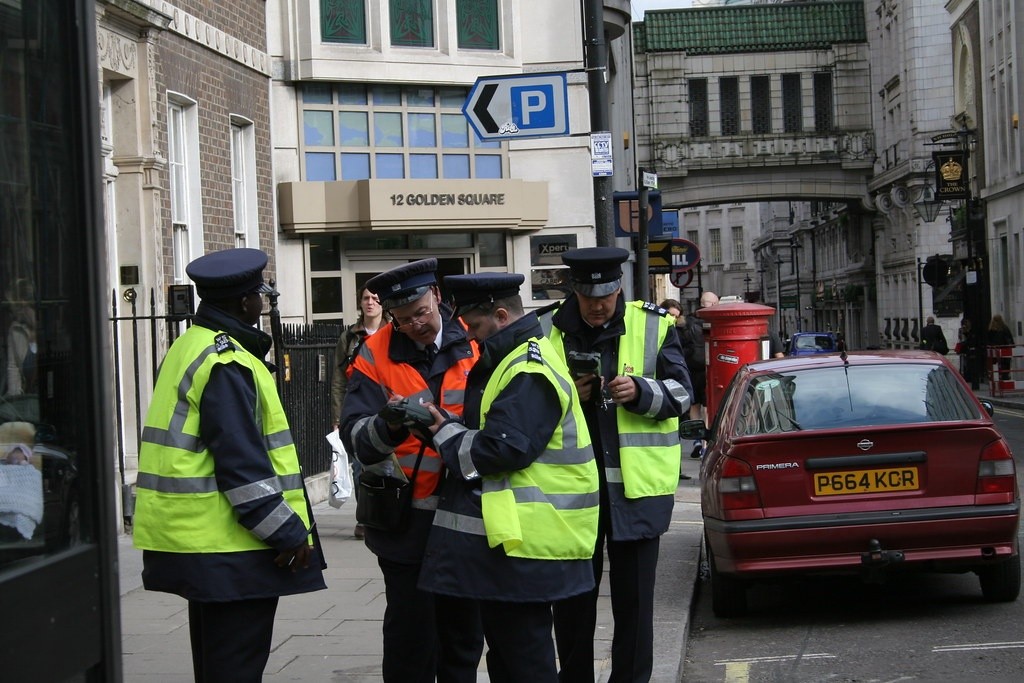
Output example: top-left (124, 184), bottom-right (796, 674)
top-left (954, 342), bottom-right (965, 354)
top-left (325, 428), bottom-right (352, 509)
top-left (356, 472), bottom-right (412, 532)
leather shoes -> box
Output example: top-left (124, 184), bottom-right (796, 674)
top-left (680, 474), bottom-right (692, 480)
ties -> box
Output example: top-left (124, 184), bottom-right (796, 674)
top-left (426, 343), bottom-right (437, 362)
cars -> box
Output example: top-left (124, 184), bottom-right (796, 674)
top-left (677, 348), bottom-right (1022, 620)
top-left (785, 331), bottom-right (839, 356)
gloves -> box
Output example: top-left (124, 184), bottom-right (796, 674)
top-left (276, 544), bottom-right (310, 568)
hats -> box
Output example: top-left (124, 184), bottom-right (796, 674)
top-left (186, 248), bottom-right (273, 301)
top-left (444, 272), bottom-right (525, 322)
top-left (560, 247), bottom-right (630, 298)
top-left (366, 258), bottom-right (437, 308)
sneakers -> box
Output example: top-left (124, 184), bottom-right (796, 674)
top-left (690, 439), bottom-right (703, 459)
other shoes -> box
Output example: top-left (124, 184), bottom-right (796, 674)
top-left (354, 524), bottom-right (364, 537)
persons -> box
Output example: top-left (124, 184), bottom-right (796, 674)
top-left (0, 275), bottom-right (73, 541)
top-left (418, 271), bottom-right (600, 683)
top-left (132, 248), bottom-right (329, 683)
top-left (957, 314), bottom-right (1016, 391)
top-left (538, 246), bottom-right (694, 683)
top-left (834, 332), bottom-right (846, 352)
top-left (659, 291), bottom-right (784, 483)
top-left (339, 258), bottom-right (485, 683)
top-left (331, 284), bottom-right (393, 540)
top-left (920, 317), bottom-right (949, 356)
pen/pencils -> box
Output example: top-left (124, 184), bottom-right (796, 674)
top-left (383, 383), bottom-right (404, 403)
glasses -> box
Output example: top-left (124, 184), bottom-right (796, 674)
top-left (391, 289), bottom-right (434, 334)
